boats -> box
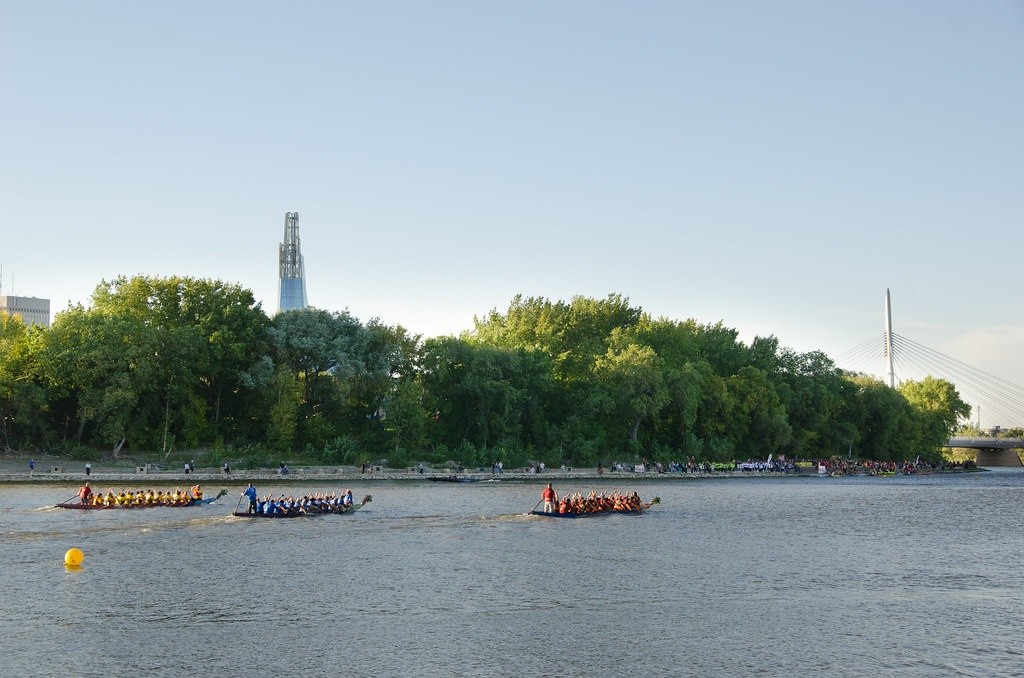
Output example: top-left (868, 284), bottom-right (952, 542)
top-left (55, 487), bottom-right (228, 510)
top-left (530, 495), bottom-right (661, 518)
top-left (232, 494), bottom-right (372, 517)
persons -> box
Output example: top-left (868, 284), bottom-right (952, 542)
top-left (280, 461), bottom-right (289, 475)
top-left (530, 460), bottom-right (545, 473)
top-left (361, 461), bottom-right (365, 473)
top-left (541, 483), bottom-right (649, 514)
top-left (184, 459), bottom-right (195, 475)
top-left (77, 481), bottom-right (202, 506)
top-left (491, 461), bottom-right (503, 474)
top-left (611, 457), bottom-right (976, 474)
top-left (84, 461), bottom-right (91, 475)
top-left (418, 462), bottom-right (423, 474)
top-left (241, 483), bottom-right (352, 515)
top-left (369, 462), bottom-right (374, 473)
top-left (29, 458), bottom-right (35, 474)
top-left (224, 461), bottom-right (232, 475)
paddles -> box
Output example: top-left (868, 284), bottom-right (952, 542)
top-left (329, 503), bottom-right (340, 513)
top-left (109, 488), bottom-right (124, 511)
top-left (310, 505), bottom-right (320, 513)
top-left (234, 490), bottom-right (246, 514)
top-left (300, 504), bottom-right (308, 515)
top-left (336, 504), bottom-right (346, 513)
top-left (55, 493), bottom-right (79, 507)
top-left (187, 490), bottom-right (195, 503)
top-left (271, 495), bottom-right (287, 514)
